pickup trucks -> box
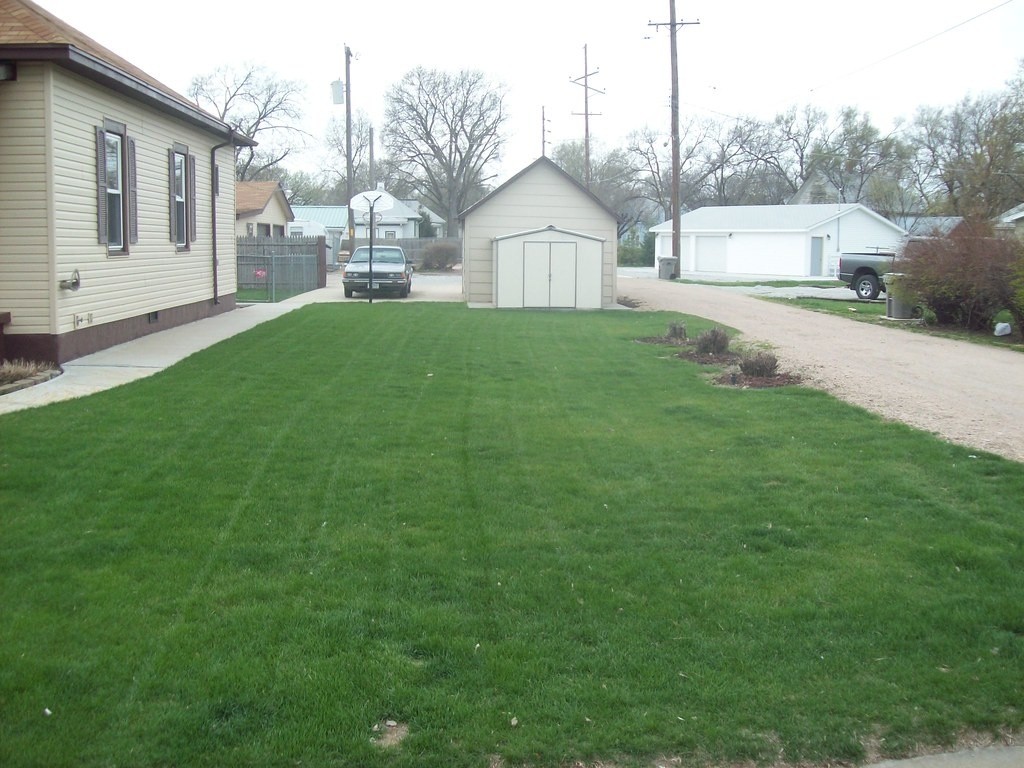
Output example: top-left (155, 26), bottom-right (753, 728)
top-left (838, 234), bottom-right (952, 302)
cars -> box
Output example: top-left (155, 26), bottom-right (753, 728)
top-left (344, 245), bottom-right (413, 299)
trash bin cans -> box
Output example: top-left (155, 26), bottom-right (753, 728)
top-left (658, 256), bottom-right (678, 280)
top-left (882, 272), bottom-right (917, 319)
